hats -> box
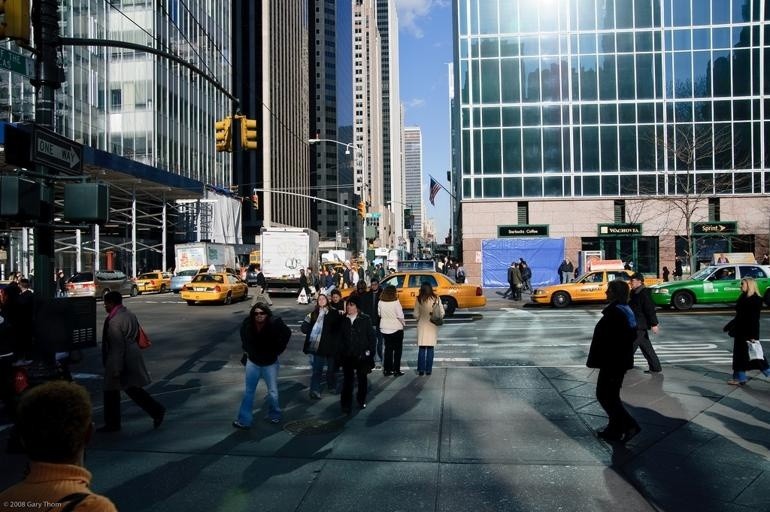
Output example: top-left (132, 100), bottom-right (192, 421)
top-left (630, 272), bottom-right (643, 281)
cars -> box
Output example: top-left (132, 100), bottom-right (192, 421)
top-left (336, 271), bottom-right (487, 316)
top-left (134, 271), bottom-right (173, 294)
top-left (646, 250), bottom-right (770, 309)
top-left (171, 267), bottom-right (208, 292)
top-left (0, 279), bottom-right (32, 295)
top-left (533, 269), bottom-right (665, 304)
top-left (180, 264), bottom-right (249, 305)
top-left (320, 255), bottom-right (365, 276)
top-left (239, 267), bottom-right (247, 282)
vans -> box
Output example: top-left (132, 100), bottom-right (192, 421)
top-left (382, 260), bottom-right (438, 275)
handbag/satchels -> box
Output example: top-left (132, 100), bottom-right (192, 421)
top-left (747, 339), bottom-right (765, 361)
top-left (430, 303), bottom-right (443, 326)
top-left (137, 326), bottom-right (152, 350)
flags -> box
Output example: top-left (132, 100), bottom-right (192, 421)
top-left (429, 180), bottom-right (443, 206)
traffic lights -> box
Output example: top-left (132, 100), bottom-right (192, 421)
top-left (252, 195), bottom-right (257, 210)
top-left (215, 122), bottom-right (226, 151)
top-left (0, 0), bottom-right (7, 37)
top-left (358, 203), bottom-right (364, 217)
top-left (246, 120), bottom-right (259, 150)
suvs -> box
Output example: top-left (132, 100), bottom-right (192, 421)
top-left (247, 263), bottom-right (261, 286)
top-left (64, 269), bottom-right (139, 299)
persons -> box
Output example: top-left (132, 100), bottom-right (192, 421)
top-left (96, 291), bottom-right (166, 433)
top-left (761, 253), bottom-right (770, 265)
top-left (0, 269), bottom-right (65, 298)
top-left (724, 277), bottom-right (770, 385)
top-left (718, 253), bottom-right (729, 263)
top-left (296, 256), bottom-right (464, 415)
top-left (0, 379), bottom-right (119, 512)
top-left (505, 257), bottom-right (533, 301)
top-left (672, 254), bottom-right (682, 281)
top-left (628, 272), bottom-right (663, 373)
top-left (234, 302), bottom-right (291, 430)
top-left (560, 257), bottom-right (573, 284)
top-left (586, 280), bottom-right (642, 445)
top-left (720, 269), bottom-right (730, 280)
top-left (250, 268), bottom-right (272, 307)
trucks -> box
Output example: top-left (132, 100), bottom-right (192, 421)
top-left (174, 241), bottom-right (240, 275)
top-left (259, 225), bottom-right (320, 293)
top-left (322, 248), bottom-right (352, 270)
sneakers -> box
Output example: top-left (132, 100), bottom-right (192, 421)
top-left (271, 416), bottom-right (281, 424)
top-left (645, 369), bottom-right (661, 373)
top-left (419, 370), bottom-right (431, 375)
top-left (309, 386), bottom-right (367, 416)
top-left (233, 417), bottom-right (249, 429)
top-left (596, 422), bottom-right (641, 448)
top-left (727, 378), bottom-right (745, 386)
top-left (96, 423), bottom-right (121, 432)
top-left (153, 405), bottom-right (165, 429)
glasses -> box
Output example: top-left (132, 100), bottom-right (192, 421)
top-left (253, 312), bottom-right (266, 317)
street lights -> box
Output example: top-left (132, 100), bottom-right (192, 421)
top-left (387, 200), bottom-right (412, 259)
top-left (334, 226), bottom-right (351, 249)
top-left (307, 137), bottom-right (369, 270)
top-left (417, 239), bottom-right (424, 260)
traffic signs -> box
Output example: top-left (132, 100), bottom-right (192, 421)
top-left (33, 128), bottom-right (85, 172)
top-left (0, 49), bottom-right (35, 79)
top-left (366, 212), bottom-right (382, 219)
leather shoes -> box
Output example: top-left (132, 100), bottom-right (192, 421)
top-left (384, 369), bottom-right (404, 377)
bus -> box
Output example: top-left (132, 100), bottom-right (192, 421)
top-left (248, 250), bottom-right (261, 265)
